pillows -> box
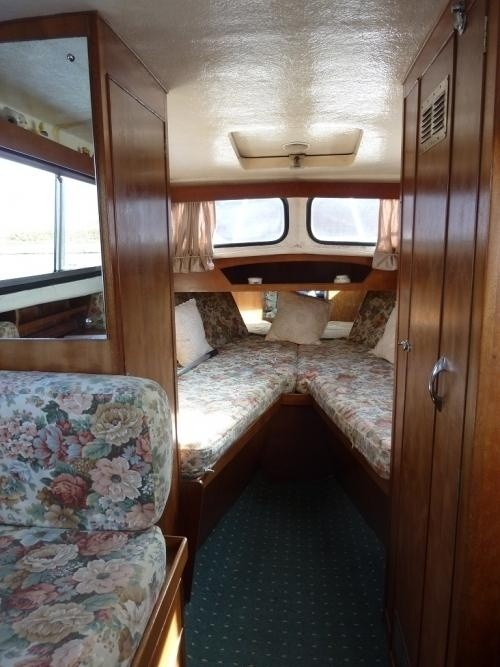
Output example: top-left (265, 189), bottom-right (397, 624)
top-left (174, 290), bottom-right (399, 366)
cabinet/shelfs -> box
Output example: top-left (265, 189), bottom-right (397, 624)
top-left (379, 0), bottom-right (500, 664)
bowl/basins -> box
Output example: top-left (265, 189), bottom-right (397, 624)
top-left (334, 274), bottom-right (351, 284)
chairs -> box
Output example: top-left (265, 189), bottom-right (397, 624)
top-left (1, 368), bottom-right (188, 665)
